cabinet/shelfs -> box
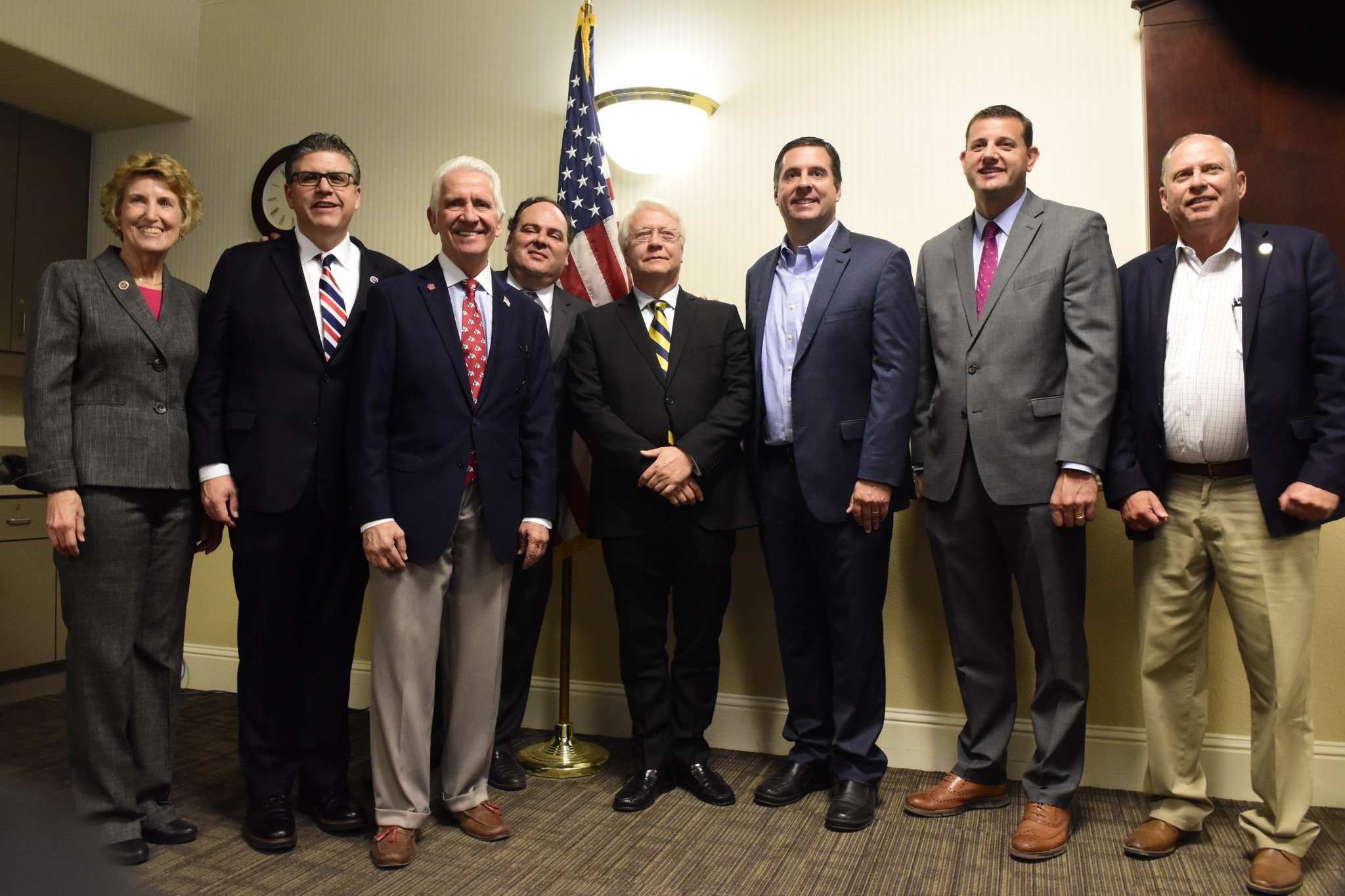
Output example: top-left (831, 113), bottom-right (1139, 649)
top-left (0, 475), bottom-right (77, 678)
top-left (0, 99), bottom-right (93, 380)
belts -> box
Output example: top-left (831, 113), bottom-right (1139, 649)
top-left (1170, 458), bottom-right (1250, 480)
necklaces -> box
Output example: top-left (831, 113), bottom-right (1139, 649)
top-left (129, 274), bottom-right (164, 286)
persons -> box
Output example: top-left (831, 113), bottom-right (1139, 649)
top-left (909, 100), bottom-right (1127, 865)
top-left (356, 153), bottom-right (559, 869)
top-left (567, 196), bottom-right (756, 812)
top-left (1100, 123), bottom-right (1344, 895)
top-left (247, 195), bottom-right (720, 789)
top-left (739, 132), bottom-right (923, 834)
top-left (181, 133), bottom-right (412, 852)
top-left (21, 150), bottom-right (228, 867)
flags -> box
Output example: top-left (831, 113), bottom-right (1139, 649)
top-left (552, 8), bottom-right (635, 541)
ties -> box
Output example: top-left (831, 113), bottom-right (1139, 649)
top-left (460, 278), bottom-right (485, 484)
top-left (975, 221), bottom-right (1003, 315)
top-left (648, 301), bottom-right (676, 447)
top-left (521, 289), bottom-right (537, 300)
top-left (316, 254), bottom-right (347, 363)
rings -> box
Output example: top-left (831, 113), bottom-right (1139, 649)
top-left (1074, 514), bottom-right (1085, 520)
top-left (871, 511), bottom-right (880, 514)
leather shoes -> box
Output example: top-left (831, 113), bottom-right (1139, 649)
top-left (1010, 802), bottom-right (1078, 860)
top-left (439, 801), bottom-right (510, 842)
top-left (108, 839), bottom-right (147, 864)
top-left (1246, 848), bottom-right (1305, 894)
top-left (904, 773), bottom-right (1011, 817)
top-left (677, 763), bottom-right (735, 806)
top-left (826, 780), bottom-right (880, 830)
top-left (754, 760), bottom-right (831, 806)
top-left (487, 741), bottom-right (526, 789)
top-left (142, 818), bottom-right (198, 844)
top-left (243, 791), bottom-right (298, 849)
top-left (297, 785), bottom-right (373, 830)
top-left (613, 770), bottom-right (679, 811)
top-left (370, 824), bottom-right (419, 868)
top-left (1121, 817), bottom-right (1185, 857)
